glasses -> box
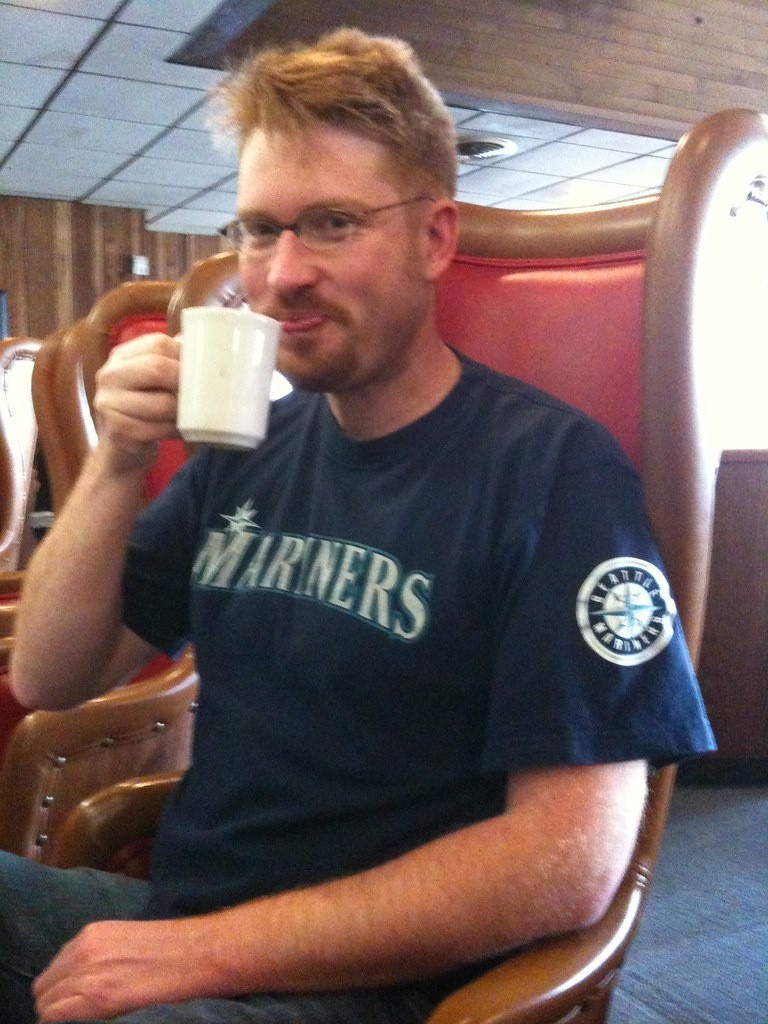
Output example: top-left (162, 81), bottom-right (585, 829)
top-left (218, 196), bottom-right (438, 254)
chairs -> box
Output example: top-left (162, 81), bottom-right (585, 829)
top-left (0, 106), bottom-right (768, 1024)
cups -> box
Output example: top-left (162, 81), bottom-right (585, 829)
top-left (173, 305), bottom-right (284, 452)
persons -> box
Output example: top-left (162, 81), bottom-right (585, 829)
top-left (0, 26), bottom-right (716, 1024)
